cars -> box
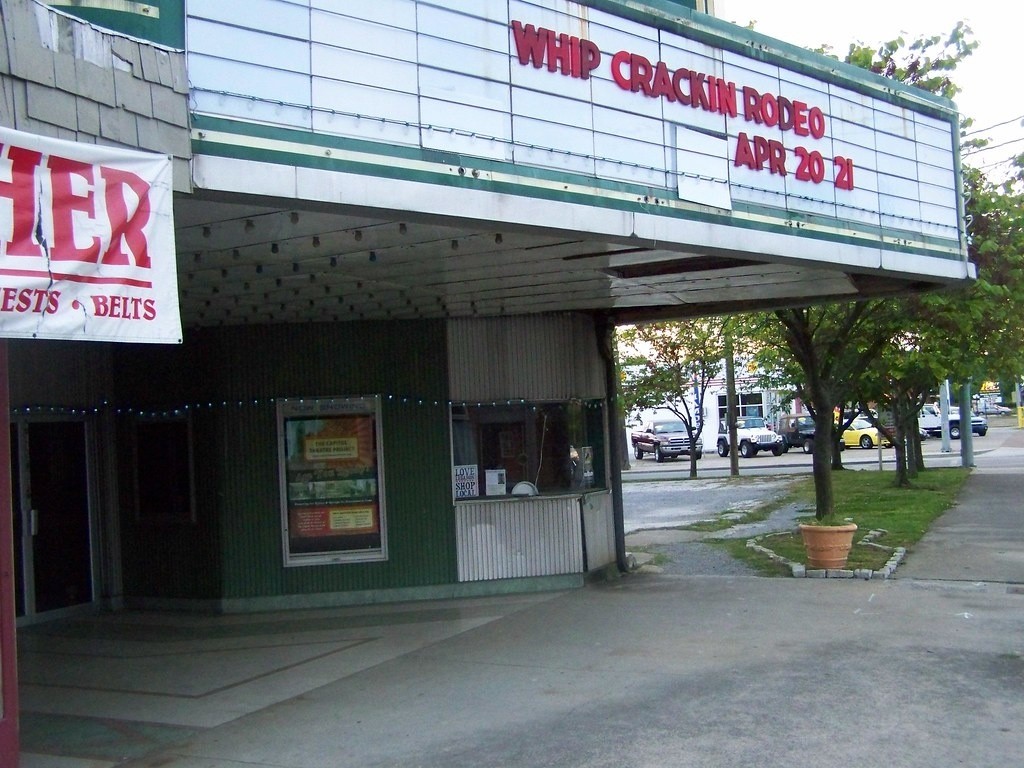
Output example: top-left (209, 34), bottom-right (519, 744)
top-left (841, 419), bottom-right (893, 450)
top-left (977, 403), bottom-right (1012, 417)
top-left (841, 407), bottom-right (931, 449)
top-left (949, 406), bottom-right (988, 436)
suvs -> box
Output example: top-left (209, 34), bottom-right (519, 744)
top-left (716, 416), bottom-right (784, 459)
top-left (779, 413), bottom-right (846, 456)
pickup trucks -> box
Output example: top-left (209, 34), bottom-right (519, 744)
top-left (915, 403), bottom-right (960, 439)
top-left (631, 419), bottom-right (703, 463)
